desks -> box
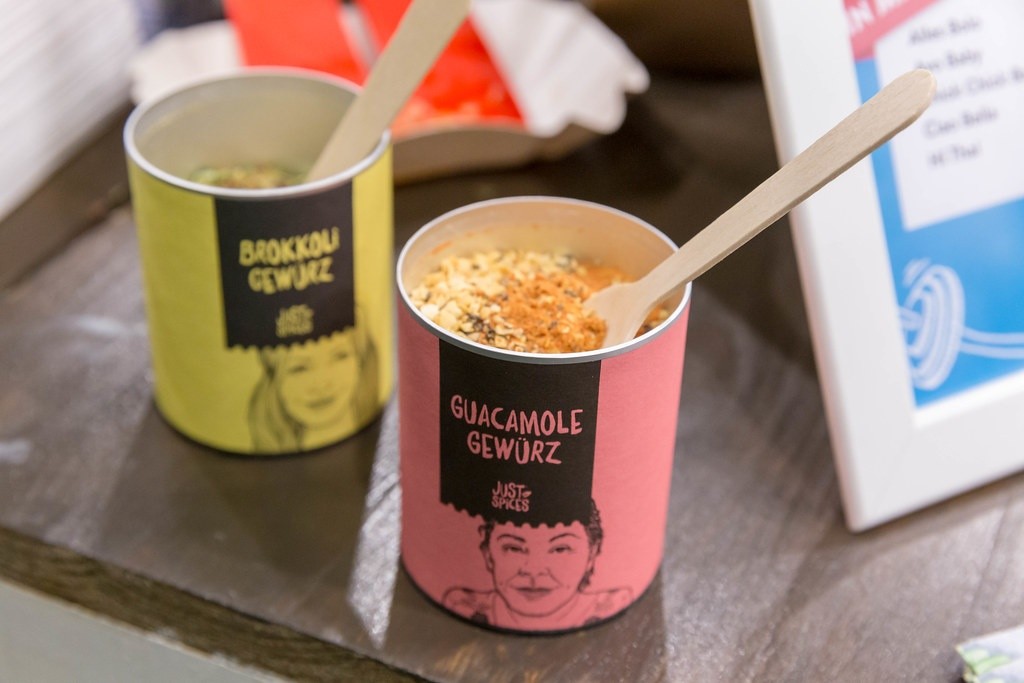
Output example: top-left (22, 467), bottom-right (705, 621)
top-left (0, 0), bottom-right (1024, 683)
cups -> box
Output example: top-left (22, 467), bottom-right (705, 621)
top-left (394, 198), bottom-right (692, 633)
top-left (125, 67), bottom-right (394, 453)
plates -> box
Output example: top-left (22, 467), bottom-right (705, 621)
top-left (123, 0), bottom-right (649, 184)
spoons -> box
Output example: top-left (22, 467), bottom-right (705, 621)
top-left (583, 72), bottom-right (933, 350)
